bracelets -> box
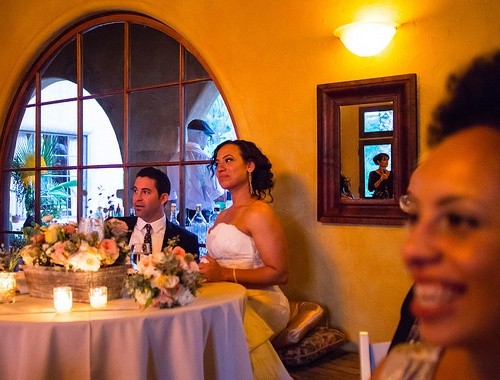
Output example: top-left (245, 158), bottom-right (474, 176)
top-left (233, 268), bottom-right (237, 283)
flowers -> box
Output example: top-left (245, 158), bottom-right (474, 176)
top-left (126, 236), bottom-right (205, 309)
top-left (18, 215), bottom-right (130, 269)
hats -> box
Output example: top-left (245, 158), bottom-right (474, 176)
top-left (187, 119), bottom-right (215, 134)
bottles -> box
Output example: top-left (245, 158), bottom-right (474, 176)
top-left (209, 205), bottom-right (219, 227)
top-left (189, 204), bottom-right (206, 255)
top-left (87, 204), bottom-right (136, 230)
top-left (168, 203), bottom-right (181, 226)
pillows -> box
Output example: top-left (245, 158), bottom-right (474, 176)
top-left (277, 299), bottom-right (324, 344)
top-left (279, 328), bottom-right (350, 366)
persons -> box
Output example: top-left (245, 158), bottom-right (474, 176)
top-left (369, 47), bottom-right (500, 380)
top-left (198, 140), bottom-right (290, 340)
top-left (167, 119), bottom-right (227, 226)
top-left (368, 152), bottom-right (393, 199)
top-left (104, 167), bottom-right (200, 264)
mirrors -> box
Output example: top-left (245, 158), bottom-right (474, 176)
top-left (318, 74), bottom-right (418, 227)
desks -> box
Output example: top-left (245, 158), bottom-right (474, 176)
top-left (0, 272), bottom-right (253, 380)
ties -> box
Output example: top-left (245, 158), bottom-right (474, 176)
top-left (142, 224), bottom-right (152, 255)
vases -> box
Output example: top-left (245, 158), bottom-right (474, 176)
top-left (24, 265), bottom-right (129, 302)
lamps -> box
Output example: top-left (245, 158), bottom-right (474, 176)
top-left (333, 20), bottom-right (400, 58)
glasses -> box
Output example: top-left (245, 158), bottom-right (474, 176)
top-left (206, 134), bottom-right (212, 141)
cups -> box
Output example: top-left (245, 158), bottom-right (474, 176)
top-left (53, 287), bottom-right (73, 312)
top-left (131, 243), bottom-right (150, 270)
top-left (90, 286), bottom-right (107, 309)
top-left (0, 272), bottom-right (16, 304)
